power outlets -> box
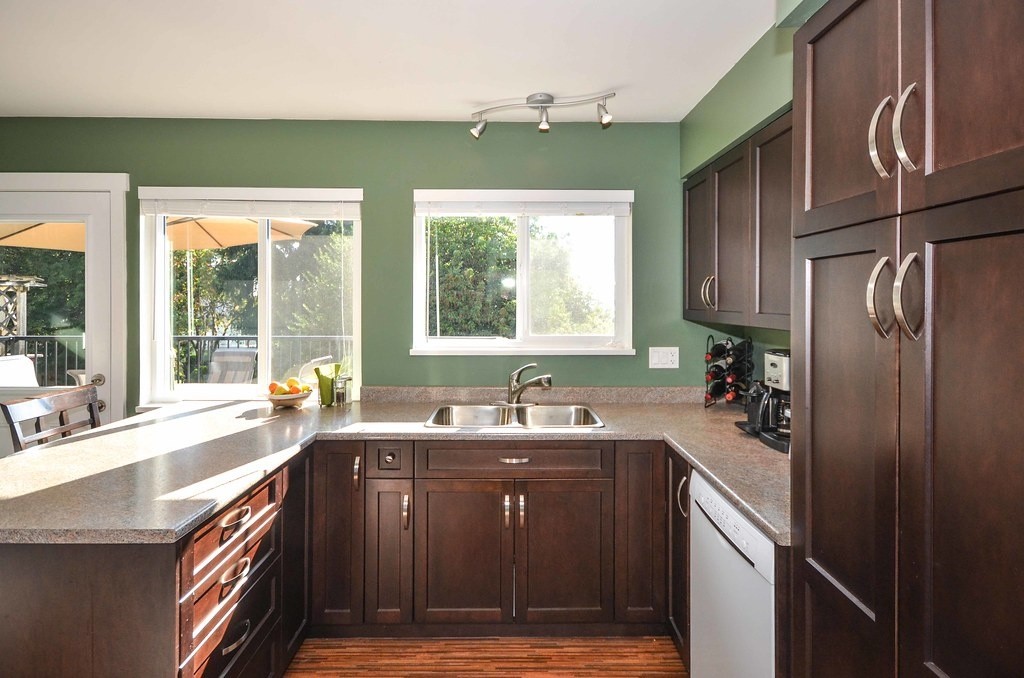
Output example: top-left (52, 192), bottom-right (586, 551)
top-left (649, 346), bottom-right (680, 369)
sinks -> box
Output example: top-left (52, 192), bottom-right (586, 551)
top-left (515, 405), bottom-right (605, 429)
top-left (424, 404), bottom-right (513, 428)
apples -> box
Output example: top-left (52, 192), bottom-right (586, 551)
top-left (275, 384), bottom-right (289, 395)
top-left (302, 386), bottom-right (312, 392)
top-left (287, 377), bottom-right (300, 387)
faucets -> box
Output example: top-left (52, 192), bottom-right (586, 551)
top-left (506, 361), bottom-right (553, 407)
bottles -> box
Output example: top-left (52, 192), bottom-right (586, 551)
top-left (333, 379), bottom-right (345, 406)
top-left (704, 339), bottom-right (734, 401)
top-left (342, 376), bottom-right (353, 403)
top-left (725, 341), bottom-right (754, 401)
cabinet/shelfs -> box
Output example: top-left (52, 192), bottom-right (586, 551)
top-left (789, 0), bottom-right (1024, 678)
top-left (307, 440), bottom-right (690, 678)
top-left (0, 450), bottom-right (308, 678)
top-left (683, 110), bottom-right (791, 332)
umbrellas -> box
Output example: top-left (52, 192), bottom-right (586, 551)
top-left (0, 217), bottom-right (318, 253)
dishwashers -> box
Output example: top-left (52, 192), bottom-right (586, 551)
top-left (689, 468), bottom-right (775, 678)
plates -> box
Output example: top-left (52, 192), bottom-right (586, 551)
top-left (264, 390), bottom-right (311, 410)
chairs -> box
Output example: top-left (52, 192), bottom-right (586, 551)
top-left (206, 347), bottom-right (258, 384)
top-left (299, 355), bottom-right (332, 381)
top-left (65, 370), bottom-right (85, 386)
top-left (0, 383), bottom-right (101, 452)
top-left (0, 355), bottom-right (39, 387)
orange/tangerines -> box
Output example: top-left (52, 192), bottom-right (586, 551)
top-left (291, 386), bottom-right (301, 394)
top-left (269, 382), bottom-right (278, 392)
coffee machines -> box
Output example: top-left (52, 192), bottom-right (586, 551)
top-left (757, 349), bottom-right (790, 454)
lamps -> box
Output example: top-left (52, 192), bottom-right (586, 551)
top-left (470, 92), bottom-right (615, 138)
